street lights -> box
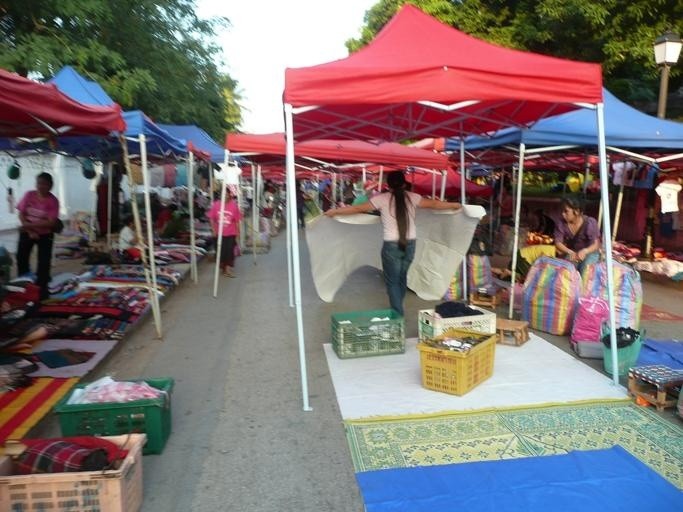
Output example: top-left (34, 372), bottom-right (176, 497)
top-left (654, 32), bottom-right (683, 118)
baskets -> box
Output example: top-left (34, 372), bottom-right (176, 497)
top-left (417, 304), bottom-right (497, 343)
top-left (415, 326), bottom-right (497, 397)
top-left (0, 432), bottom-right (148, 512)
top-left (330, 307), bottom-right (406, 359)
top-left (52, 376), bottom-right (175, 456)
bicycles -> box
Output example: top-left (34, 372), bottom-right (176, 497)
top-left (266, 195), bottom-right (286, 231)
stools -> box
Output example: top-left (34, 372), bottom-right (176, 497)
top-left (469, 286), bottom-right (502, 310)
top-left (627, 364), bottom-right (683, 414)
top-left (496, 318), bottom-right (529, 345)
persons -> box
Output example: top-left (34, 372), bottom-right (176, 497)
top-left (0, 245), bottom-right (12, 276)
top-left (322, 171), bottom-right (464, 319)
top-left (0, 268), bottom-right (48, 353)
top-left (15, 172), bottom-right (58, 300)
top-left (148, 177), bottom-right (356, 229)
top-left (154, 204), bottom-right (186, 240)
top-left (207, 188), bottom-right (242, 278)
top-left (520, 205), bottom-right (554, 236)
top-left (487, 183), bottom-right (513, 242)
top-left (118, 217), bottom-right (148, 262)
top-left (554, 192), bottom-right (601, 274)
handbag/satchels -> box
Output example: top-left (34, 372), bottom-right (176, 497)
top-left (519, 254), bottom-right (582, 336)
top-left (569, 251), bottom-right (643, 348)
top-left (597, 316), bottom-right (649, 377)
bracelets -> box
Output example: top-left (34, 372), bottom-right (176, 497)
top-left (566, 250), bottom-right (574, 256)
top-left (582, 248), bottom-right (591, 254)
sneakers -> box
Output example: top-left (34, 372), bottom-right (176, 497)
top-left (224, 271), bottom-right (238, 281)
